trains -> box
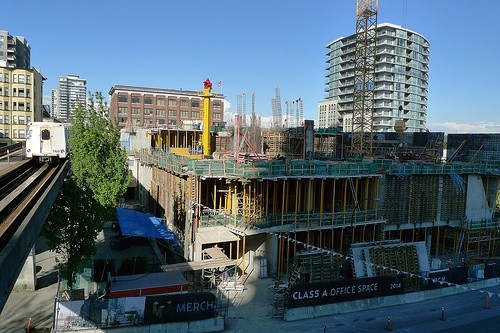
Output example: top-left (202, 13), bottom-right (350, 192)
top-left (25, 121), bottom-right (73, 164)
